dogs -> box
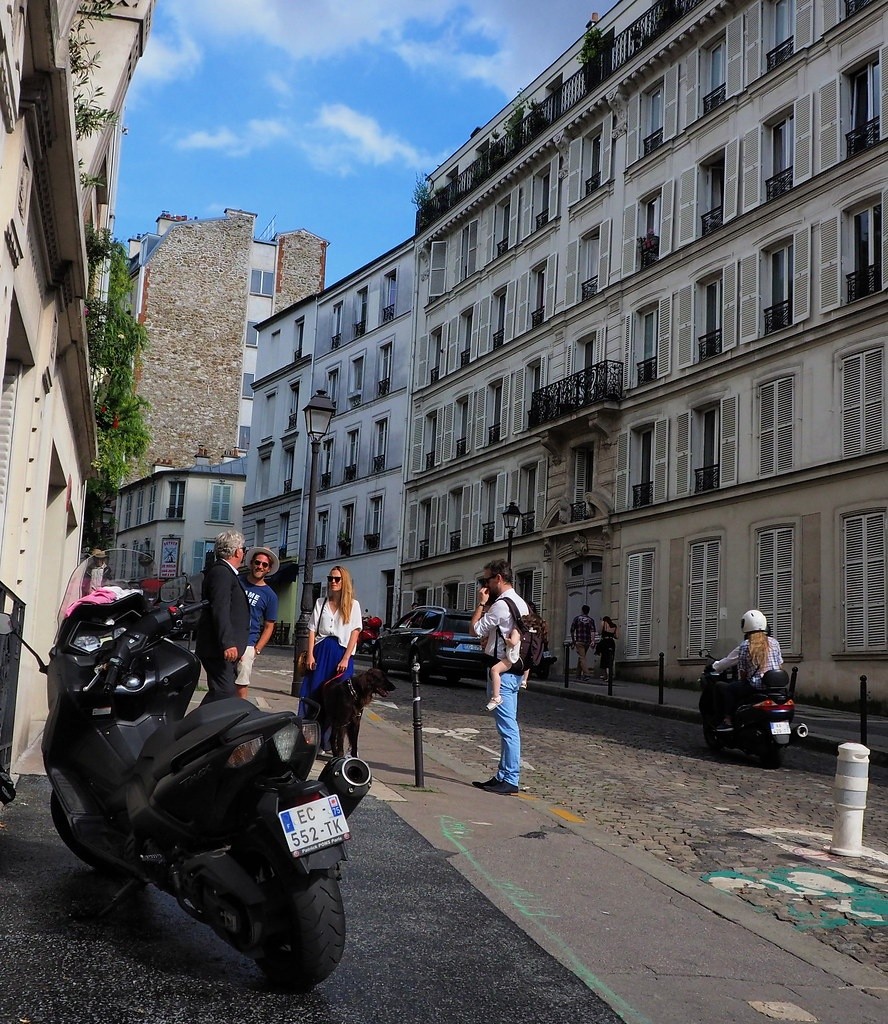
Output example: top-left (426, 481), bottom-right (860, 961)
top-left (305, 668), bottom-right (397, 757)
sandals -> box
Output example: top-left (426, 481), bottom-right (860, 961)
top-left (486, 696), bottom-right (503, 712)
top-left (520, 680), bottom-right (527, 689)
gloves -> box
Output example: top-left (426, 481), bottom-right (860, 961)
top-left (704, 664), bottom-right (716, 673)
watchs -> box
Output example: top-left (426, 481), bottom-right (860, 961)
top-left (477, 603), bottom-right (484, 607)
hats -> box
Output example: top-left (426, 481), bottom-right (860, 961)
top-left (245, 547), bottom-right (280, 577)
top-left (88, 549), bottom-right (106, 559)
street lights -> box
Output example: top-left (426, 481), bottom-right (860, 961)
top-left (290, 389), bottom-right (339, 696)
top-left (501, 501), bottom-right (522, 582)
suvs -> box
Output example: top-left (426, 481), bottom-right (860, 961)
top-left (371, 605), bottom-right (491, 683)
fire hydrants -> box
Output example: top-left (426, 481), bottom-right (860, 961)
top-left (291, 611), bottom-right (313, 698)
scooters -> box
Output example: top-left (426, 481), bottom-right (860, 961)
top-left (357, 609), bottom-right (382, 655)
top-left (698, 648), bottom-right (810, 768)
top-left (126, 576), bottom-right (198, 638)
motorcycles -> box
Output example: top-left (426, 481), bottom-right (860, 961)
top-left (0, 547), bottom-right (374, 993)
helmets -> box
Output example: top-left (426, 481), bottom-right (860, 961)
top-left (741, 610), bottom-right (768, 633)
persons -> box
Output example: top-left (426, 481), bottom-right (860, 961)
top-left (82, 552), bottom-right (112, 597)
top-left (594, 616), bottom-right (619, 682)
top-left (232, 546), bottom-right (278, 700)
top-left (297, 567), bottom-right (363, 756)
top-left (470, 559), bottom-right (529, 796)
top-left (570, 605), bottom-right (596, 680)
top-left (412, 603), bottom-right (418, 610)
top-left (706, 609), bottom-right (784, 731)
top-left (195, 531), bottom-right (250, 707)
top-left (485, 614), bottom-right (548, 712)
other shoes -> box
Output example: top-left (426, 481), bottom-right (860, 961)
top-left (345, 749), bottom-right (360, 760)
top-left (483, 780), bottom-right (518, 796)
top-left (712, 722), bottom-right (734, 732)
top-left (472, 777), bottom-right (501, 789)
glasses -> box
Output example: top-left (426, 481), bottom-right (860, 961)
top-left (232, 547), bottom-right (246, 553)
top-left (327, 575), bottom-right (341, 583)
top-left (483, 576), bottom-right (497, 584)
top-left (253, 559), bottom-right (270, 569)
